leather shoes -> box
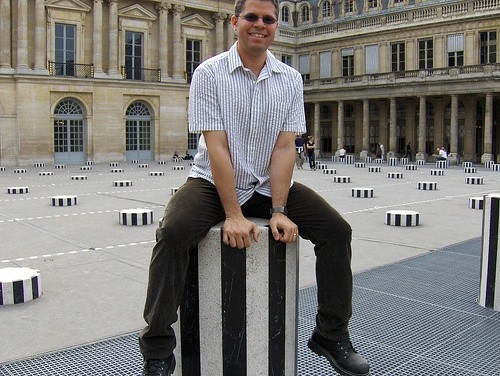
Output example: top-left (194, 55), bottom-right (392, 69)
top-left (307, 327), bottom-right (370, 376)
top-left (142, 352), bottom-right (176, 376)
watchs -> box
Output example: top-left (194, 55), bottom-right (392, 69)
top-left (270, 206), bottom-right (288, 216)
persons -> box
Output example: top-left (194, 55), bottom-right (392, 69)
top-left (184, 150), bottom-right (194, 160)
top-left (307, 136), bottom-right (317, 171)
top-left (339, 146), bottom-right (346, 158)
top-left (378, 142), bottom-right (385, 162)
top-left (138, 0), bottom-right (370, 376)
top-left (173, 151), bottom-right (180, 159)
top-left (295, 134), bottom-right (306, 169)
top-left (404, 141), bottom-right (413, 161)
top-left (441, 147), bottom-right (447, 157)
top-left (375, 144), bottom-right (382, 159)
top-left (432, 146), bottom-right (448, 161)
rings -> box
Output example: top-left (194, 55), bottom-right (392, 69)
top-left (293, 233), bottom-right (297, 236)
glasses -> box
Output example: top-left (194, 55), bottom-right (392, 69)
top-left (237, 12), bottom-right (277, 24)
